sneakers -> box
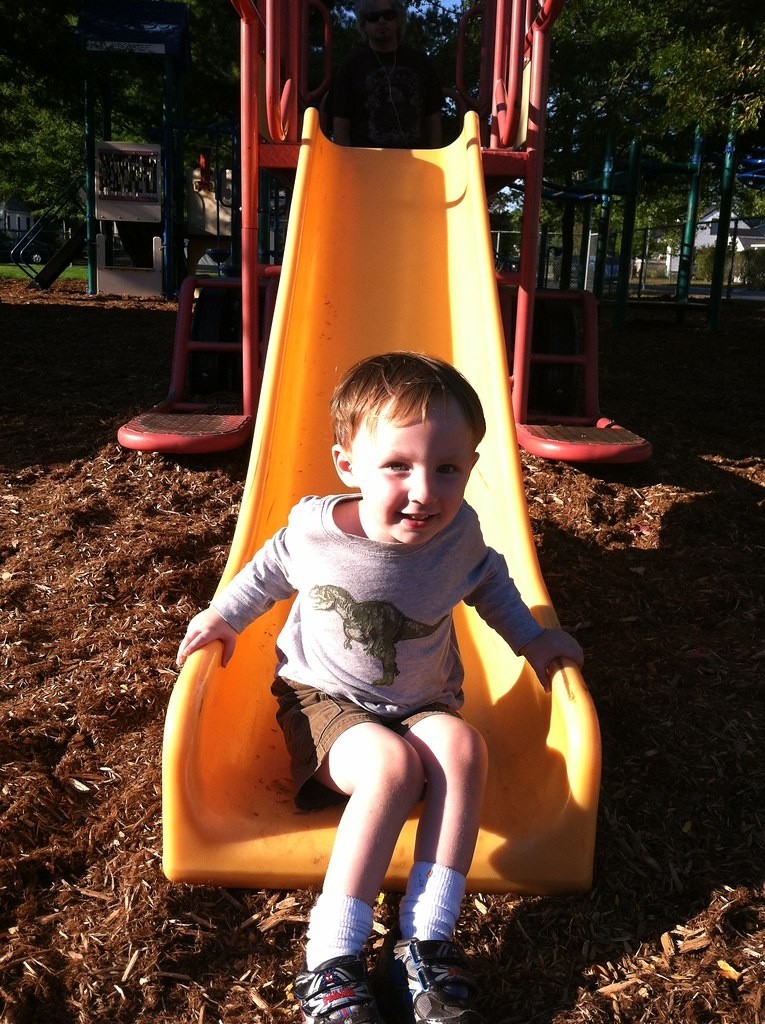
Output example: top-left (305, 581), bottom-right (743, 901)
top-left (292, 928), bottom-right (489, 1024)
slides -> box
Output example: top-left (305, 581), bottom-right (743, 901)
top-left (163, 107), bottom-right (598, 886)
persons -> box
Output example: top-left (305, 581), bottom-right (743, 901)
top-left (331, 1), bottom-right (447, 149)
top-left (176, 352), bottom-right (584, 1024)
